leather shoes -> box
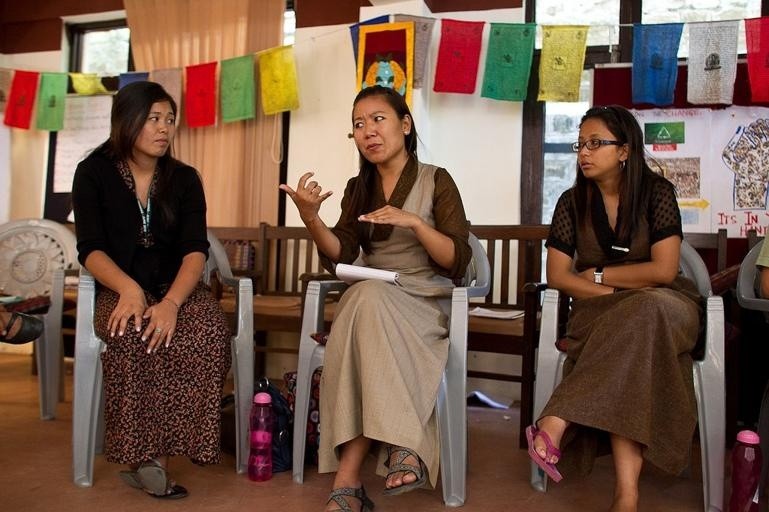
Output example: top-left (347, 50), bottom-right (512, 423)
top-left (119, 458), bottom-right (189, 498)
top-left (1, 313), bottom-right (44, 345)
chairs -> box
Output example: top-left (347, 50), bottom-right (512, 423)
top-left (528, 240), bottom-right (728, 511)
top-left (2, 217), bottom-right (82, 421)
top-left (735, 227), bottom-right (769, 484)
top-left (70, 223), bottom-right (257, 488)
top-left (289, 229), bottom-right (492, 510)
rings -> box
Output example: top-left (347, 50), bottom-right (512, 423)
top-left (155, 328), bottom-right (163, 334)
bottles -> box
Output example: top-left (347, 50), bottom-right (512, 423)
top-left (248, 392), bottom-right (274, 480)
top-left (729, 430), bottom-right (761, 511)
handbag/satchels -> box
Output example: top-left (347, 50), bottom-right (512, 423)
top-left (280, 363), bottom-right (324, 465)
top-left (222, 371), bottom-right (293, 475)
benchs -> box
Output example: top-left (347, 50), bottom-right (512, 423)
top-left (33, 222), bottom-right (764, 449)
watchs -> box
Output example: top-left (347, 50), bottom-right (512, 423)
top-left (593, 264), bottom-right (605, 285)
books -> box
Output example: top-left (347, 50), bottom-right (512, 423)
top-left (335, 262), bottom-right (405, 288)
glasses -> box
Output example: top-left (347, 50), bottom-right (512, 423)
top-left (571, 139), bottom-right (620, 152)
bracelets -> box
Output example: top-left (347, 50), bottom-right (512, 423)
top-left (162, 298), bottom-right (179, 311)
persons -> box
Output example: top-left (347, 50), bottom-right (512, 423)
top-left (278, 84), bottom-right (473, 511)
top-left (72, 81), bottom-right (232, 501)
top-left (526, 104), bottom-right (707, 512)
top-left (754, 227), bottom-right (769, 299)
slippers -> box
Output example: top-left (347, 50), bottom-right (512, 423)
top-left (526, 425), bottom-right (563, 484)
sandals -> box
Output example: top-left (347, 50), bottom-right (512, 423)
top-left (381, 446), bottom-right (426, 496)
top-left (324, 484), bottom-right (375, 512)
